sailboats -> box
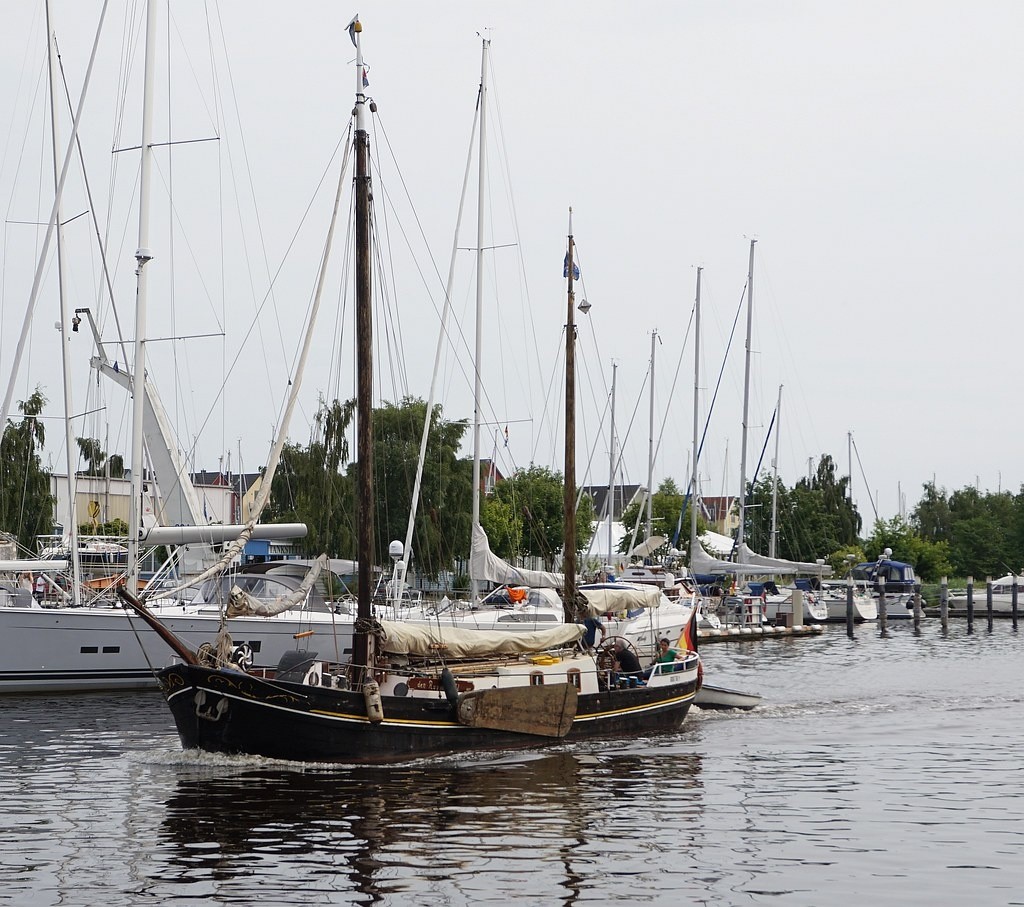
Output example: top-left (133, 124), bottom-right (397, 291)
top-left (0, 0), bottom-right (918, 690)
top-left (100, 13), bottom-right (706, 763)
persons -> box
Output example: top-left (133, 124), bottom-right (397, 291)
top-left (610, 639), bottom-right (643, 685)
top-left (18, 571), bottom-right (46, 605)
top-left (53, 573), bottom-right (63, 596)
top-left (643, 638), bottom-right (685, 681)
top-left (574, 606), bottom-right (607, 651)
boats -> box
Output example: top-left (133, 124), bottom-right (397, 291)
top-left (949, 575), bottom-right (1024, 613)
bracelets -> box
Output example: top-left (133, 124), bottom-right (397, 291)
top-left (602, 635), bottom-right (605, 637)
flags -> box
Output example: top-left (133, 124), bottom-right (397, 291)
top-left (679, 607), bottom-right (703, 674)
